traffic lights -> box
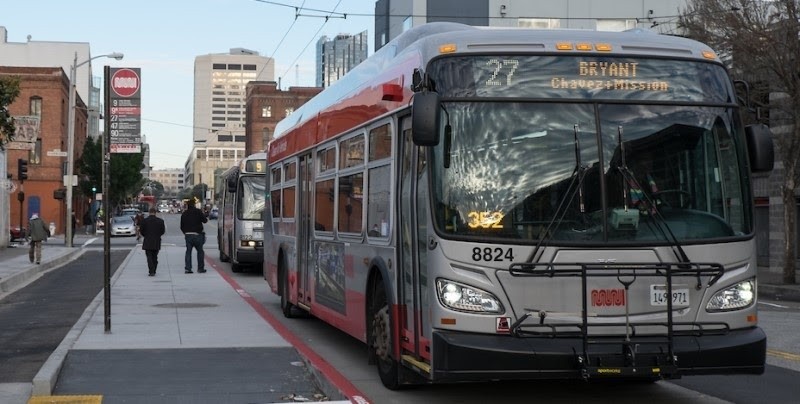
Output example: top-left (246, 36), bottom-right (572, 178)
top-left (17, 158), bottom-right (29, 185)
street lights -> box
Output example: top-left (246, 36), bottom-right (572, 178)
top-left (64, 49), bottom-right (125, 247)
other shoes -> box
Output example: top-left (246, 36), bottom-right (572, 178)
top-left (184, 269), bottom-right (194, 273)
top-left (198, 269), bottom-right (208, 273)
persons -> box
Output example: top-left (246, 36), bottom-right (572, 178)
top-left (139, 208), bottom-right (166, 276)
top-left (72, 209), bottom-right (79, 247)
top-left (180, 200), bottom-right (208, 273)
top-left (83, 209), bottom-right (92, 234)
top-left (135, 213), bottom-right (145, 239)
top-left (25, 210), bottom-right (51, 263)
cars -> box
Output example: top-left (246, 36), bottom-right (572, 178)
top-left (209, 208), bottom-right (219, 220)
top-left (10, 225), bottom-right (26, 242)
top-left (110, 216), bottom-right (136, 237)
top-left (120, 196), bottom-right (182, 221)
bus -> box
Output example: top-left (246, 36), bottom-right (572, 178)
top-left (260, 19), bottom-right (776, 393)
top-left (213, 152), bottom-right (264, 275)
